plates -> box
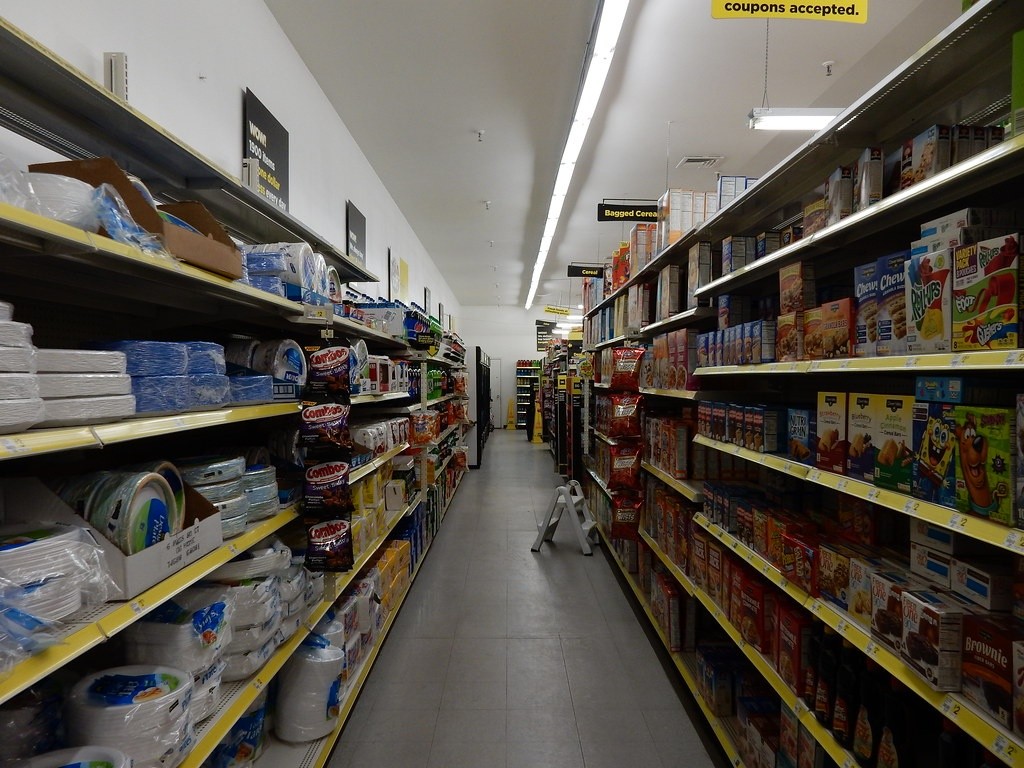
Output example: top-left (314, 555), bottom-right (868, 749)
top-left (1, 174), bottom-right (345, 768)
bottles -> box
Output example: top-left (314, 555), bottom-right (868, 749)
top-left (516, 360), bottom-right (540, 423)
top-left (344, 291), bottom-right (466, 470)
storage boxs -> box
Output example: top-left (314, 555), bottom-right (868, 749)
top-left (357, 299), bottom-right (448, 614)
top-left (554, 30), bottom-right (1023, 768)
top-left (27, 158), bottom-right (246, 282)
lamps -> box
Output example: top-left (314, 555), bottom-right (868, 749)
top-left (526, 0), bottom-right (631, 308)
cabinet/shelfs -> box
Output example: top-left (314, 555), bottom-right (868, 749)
top-left (578, 222), bottom-right (700, 729)
top-left (384, 325), bottom-right (468, 580)
top-left (512, 330), bottom-right (585, 479)
top-left (0, 14), bottom-right (258, 768)
top-left (155, 168), bottom-right (380, 767)
top-left (344, 284), bottom-right (412, 650)
top-left (804, 0), bottom-right (1024, 768)
top-left (694, 137), bottom-right (881, 768)
